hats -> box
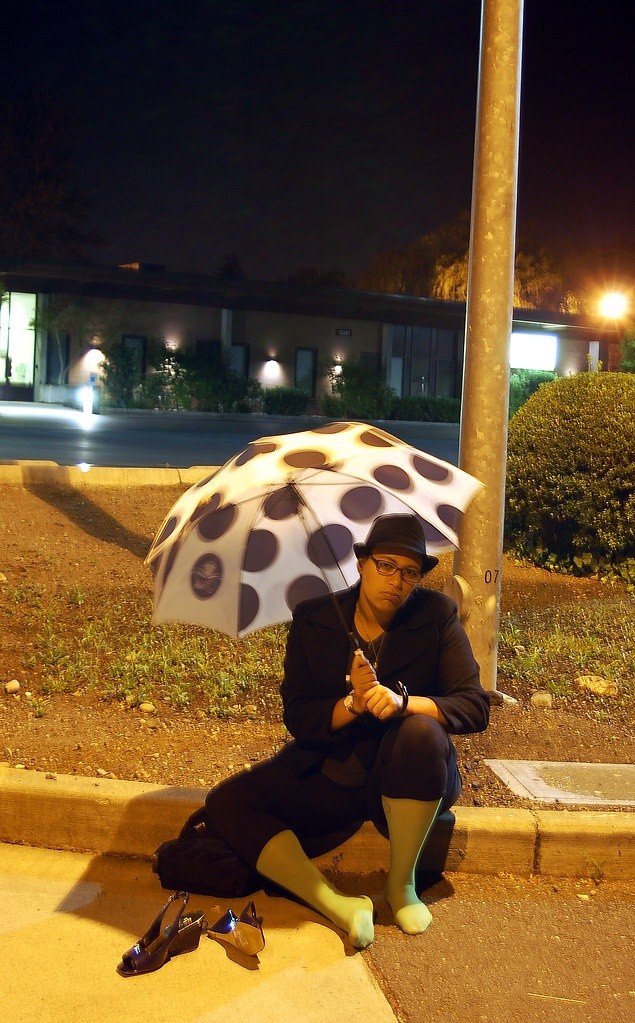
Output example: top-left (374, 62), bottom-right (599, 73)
top-left (353, 513), bottom-right (440, 573)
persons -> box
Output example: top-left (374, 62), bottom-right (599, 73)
top-left (204, 513), bottom-right (490, 947)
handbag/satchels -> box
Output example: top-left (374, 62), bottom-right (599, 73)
top-left (151, 807), bottom-right (271, 898)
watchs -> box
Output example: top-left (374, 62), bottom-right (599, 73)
top-left (343, 688), bottom-right (362, 716)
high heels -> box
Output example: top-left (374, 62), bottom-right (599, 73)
top-left (207, 900), bottom-right (266, 955)
top-left (116, 892), bottom-right (205, 977)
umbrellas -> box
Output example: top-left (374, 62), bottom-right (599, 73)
top-left (142, 420), bottom-right (488, 718)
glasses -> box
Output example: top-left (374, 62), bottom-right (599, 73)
top-left (368, 554), bottom-right (424, 584)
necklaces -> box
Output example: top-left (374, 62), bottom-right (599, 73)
top-left (355, 603), bottom-right (386, 670)
